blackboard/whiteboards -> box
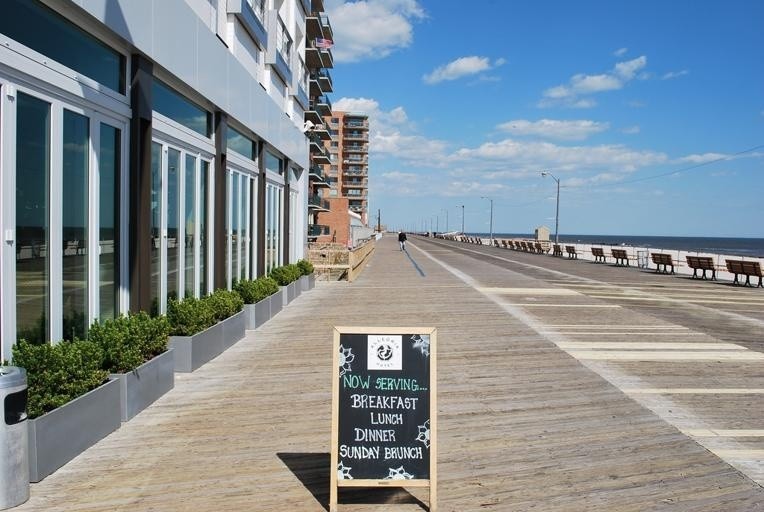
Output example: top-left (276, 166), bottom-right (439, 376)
top-left (331, 326), bottom-right (437, 488)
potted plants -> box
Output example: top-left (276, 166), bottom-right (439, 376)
top-left (85, 310), bottom-right (175, 423)
top-left (161, 287), bottom-right (246, 374)
top-left (229, 272), bottom-right (284, 330)
top-left (2, 336), bottom-right (122, 484)
top-left (266, 258), bottom-right (315, 306)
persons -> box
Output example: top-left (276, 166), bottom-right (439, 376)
top-left (398, 230), bottom-right (407, 252)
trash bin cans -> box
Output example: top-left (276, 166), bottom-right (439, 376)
top-left (2, 366), bottom-right (30, 511)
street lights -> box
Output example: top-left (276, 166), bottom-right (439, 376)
top-left (541, 170), bottom-right (561, 245)
top-left (439, 195), bottom-right (493, 239)
